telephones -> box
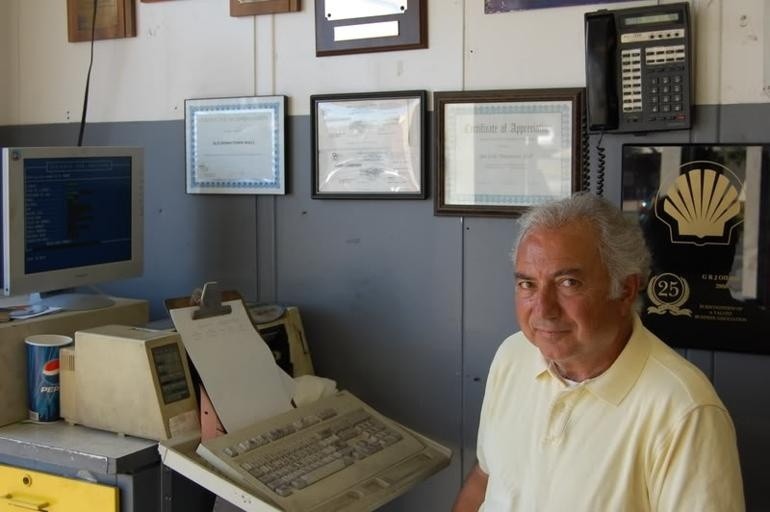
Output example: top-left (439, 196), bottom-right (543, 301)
top-left (584, 2), bottom-right (692, 135)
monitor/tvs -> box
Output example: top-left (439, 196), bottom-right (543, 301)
top-left (2, 145), bottom-right (145, 311)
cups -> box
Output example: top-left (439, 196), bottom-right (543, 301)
top-left (24, 334), bottom-right (75, 424)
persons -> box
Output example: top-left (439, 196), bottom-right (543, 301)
top-left (451, 190), bottom-right (747, 511)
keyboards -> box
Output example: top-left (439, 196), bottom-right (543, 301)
top-left (197, 388), bottom-right (424, 512)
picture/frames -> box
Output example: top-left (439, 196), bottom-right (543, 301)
top-left (227, 0), bottom-right (299, 16)
top-left (314, 0), bottom-right (429, 58)
top-left (183, 96), bottom-right (287, 196)
top-left (430, 87), bottom-right (586, 220)
top-left (616, 143), bottom-right (770, 355)
top-left (308, 90), bottom-right (428, 200)
